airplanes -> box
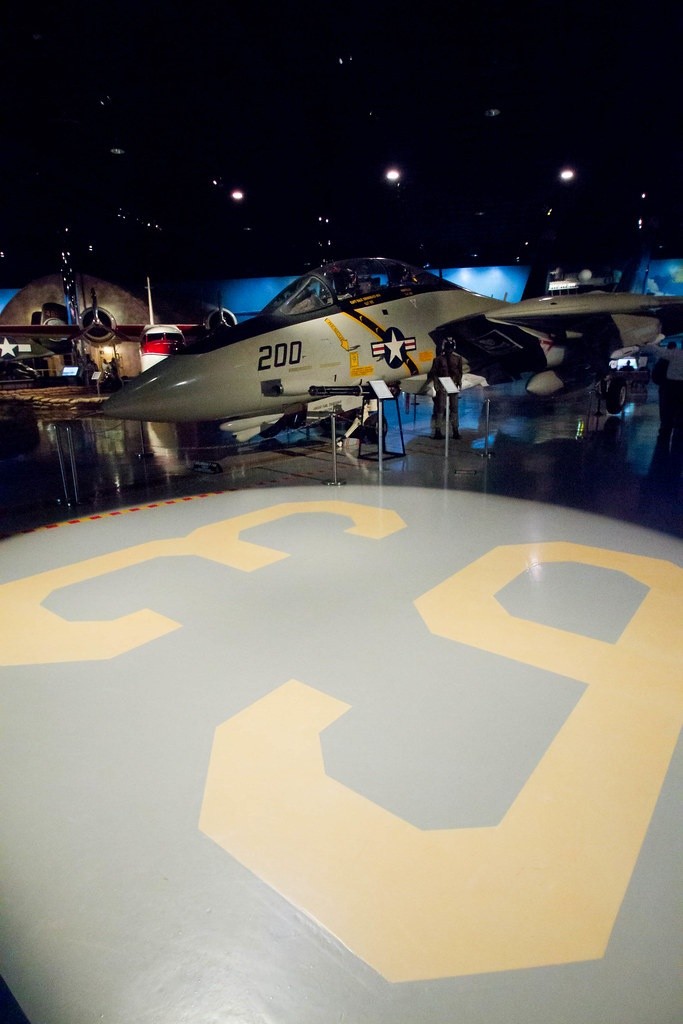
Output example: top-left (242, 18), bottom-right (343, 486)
top-left (1, 273), bottom-right (270, 395)
top-left (92, 257), bottom-right (683, 444)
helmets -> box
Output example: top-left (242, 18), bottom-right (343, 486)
top-left (442, 337), bottom-right (456, 349)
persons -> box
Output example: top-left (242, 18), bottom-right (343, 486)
top-left (90, 358), bottom-right (98, 372)
top-left (652, 342), bottom-right (677, 420)
top-left (109, 357), bottom-right (118, 375)
top-left (430, 341), bottom-right (463, 440)
top-left (642, 339), bottom-right (683, 442)
top-left (102, 359), bottom-right (112, 376)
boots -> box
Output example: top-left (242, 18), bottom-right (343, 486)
top-left (435, 428), bottom-right (443, 438)
top-left (453, 427), bottom-right (463, 439)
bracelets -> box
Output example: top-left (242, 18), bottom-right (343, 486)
top-left (644, 342), bottom-right (648, 345)
